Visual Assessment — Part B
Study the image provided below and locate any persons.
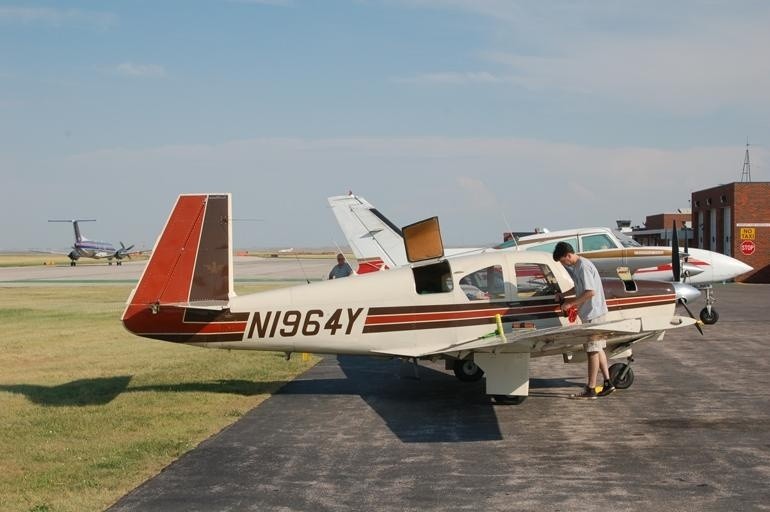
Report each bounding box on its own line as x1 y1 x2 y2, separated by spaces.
329 254 352 280
553 241 615 400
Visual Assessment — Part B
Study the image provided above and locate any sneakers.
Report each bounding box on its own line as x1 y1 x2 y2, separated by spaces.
574 386 597 400
598 379 615 396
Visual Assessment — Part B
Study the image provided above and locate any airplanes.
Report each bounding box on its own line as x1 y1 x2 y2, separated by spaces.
34 219 152 266
